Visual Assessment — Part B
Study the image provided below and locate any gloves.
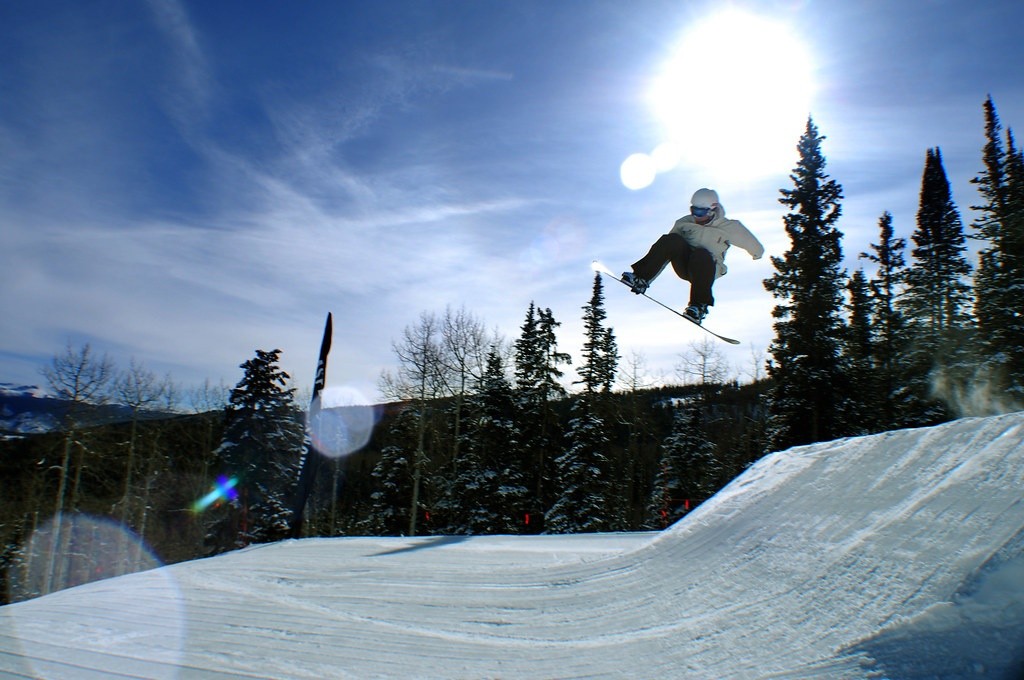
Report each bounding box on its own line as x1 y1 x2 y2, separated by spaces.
752 248 762 260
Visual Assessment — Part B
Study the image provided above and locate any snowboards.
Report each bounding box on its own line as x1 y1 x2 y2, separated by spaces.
593 261 740 347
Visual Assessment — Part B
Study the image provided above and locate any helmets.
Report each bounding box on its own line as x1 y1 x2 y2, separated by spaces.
691 188 719 209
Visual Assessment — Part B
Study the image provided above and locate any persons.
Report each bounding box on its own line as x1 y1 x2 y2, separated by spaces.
621 188 764 324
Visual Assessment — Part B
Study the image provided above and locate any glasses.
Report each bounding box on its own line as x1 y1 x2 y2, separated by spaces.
690 207 712 217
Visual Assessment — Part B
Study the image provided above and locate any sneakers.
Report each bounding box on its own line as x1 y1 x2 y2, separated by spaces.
683 303 708 324
622 272 649 294
